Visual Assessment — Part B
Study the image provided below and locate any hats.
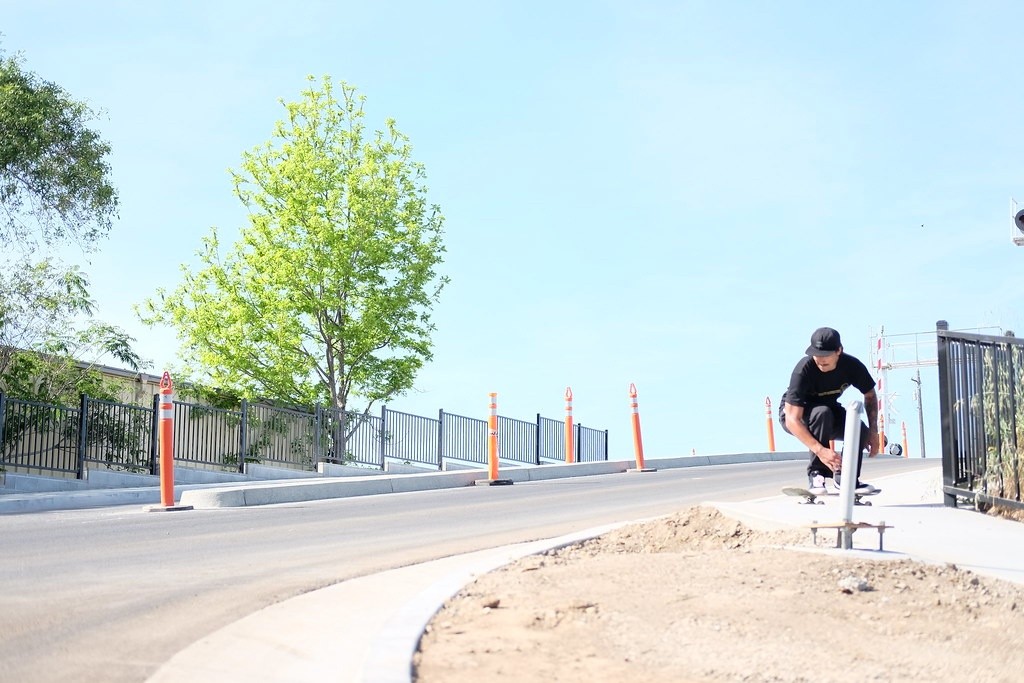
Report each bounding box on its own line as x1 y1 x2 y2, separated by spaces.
805 327 840 356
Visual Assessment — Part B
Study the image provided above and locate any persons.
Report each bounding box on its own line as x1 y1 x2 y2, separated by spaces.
779 327 881 494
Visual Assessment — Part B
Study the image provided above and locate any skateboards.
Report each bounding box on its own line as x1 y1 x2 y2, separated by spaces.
781 486 882 506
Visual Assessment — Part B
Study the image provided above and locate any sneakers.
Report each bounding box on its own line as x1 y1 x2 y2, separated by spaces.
809 471 828 494
834 479 875 494
